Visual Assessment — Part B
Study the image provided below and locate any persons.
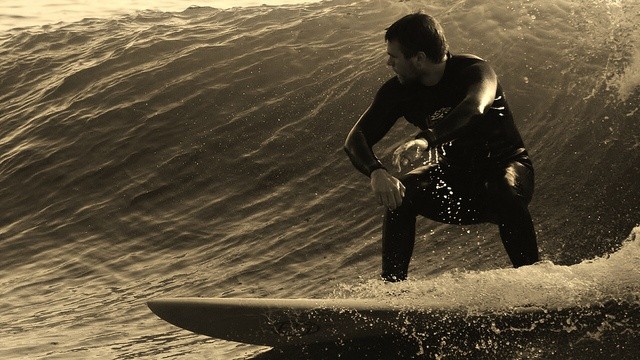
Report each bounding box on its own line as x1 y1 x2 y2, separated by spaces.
344 13 539 282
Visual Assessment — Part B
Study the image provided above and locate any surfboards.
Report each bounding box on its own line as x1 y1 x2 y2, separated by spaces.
147 298 640 360
